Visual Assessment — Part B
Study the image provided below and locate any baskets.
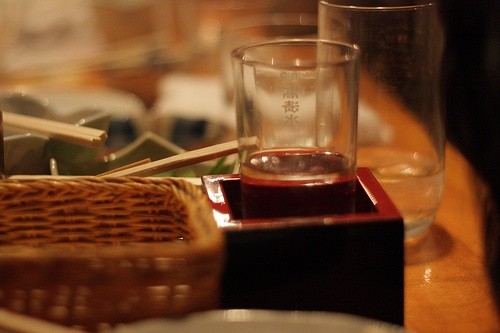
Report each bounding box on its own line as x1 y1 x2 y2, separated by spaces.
0 174 225 333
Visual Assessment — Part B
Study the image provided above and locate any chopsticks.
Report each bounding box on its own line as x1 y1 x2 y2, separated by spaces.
99 136 259 177
0 110 108 147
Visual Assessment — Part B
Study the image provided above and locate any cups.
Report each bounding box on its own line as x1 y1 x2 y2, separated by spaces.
315 1 445 237
231 38 361 219
